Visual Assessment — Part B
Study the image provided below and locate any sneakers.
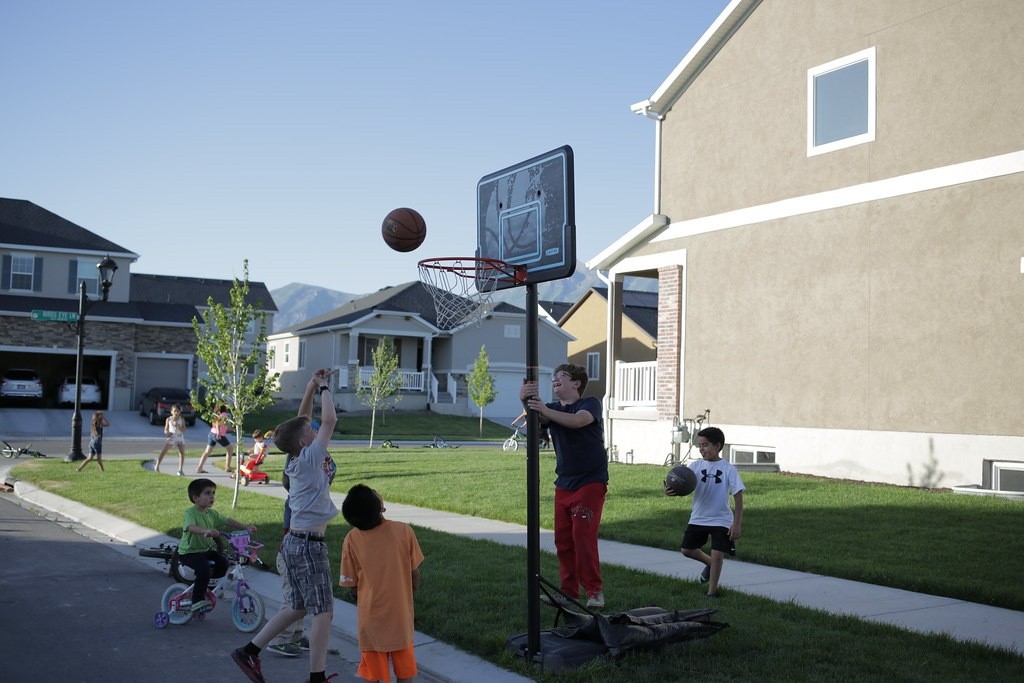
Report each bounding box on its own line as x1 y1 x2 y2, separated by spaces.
295 638 310 650
266 644 300 656
231 647 265 683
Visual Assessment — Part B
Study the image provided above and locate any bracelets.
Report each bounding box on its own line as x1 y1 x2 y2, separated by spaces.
319 386 330 394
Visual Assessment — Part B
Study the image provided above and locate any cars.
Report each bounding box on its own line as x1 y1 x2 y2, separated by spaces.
140 386 196 426
56 376 104 409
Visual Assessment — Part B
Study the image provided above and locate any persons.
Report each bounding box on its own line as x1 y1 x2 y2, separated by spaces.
177 479 257 613
197 399 233 472
512 410 550 448
76 411 110 471
663 427 746 598
229 368 340 683
519 364 610 608
154 404 185 475
246 429 269 469
339 484 425 683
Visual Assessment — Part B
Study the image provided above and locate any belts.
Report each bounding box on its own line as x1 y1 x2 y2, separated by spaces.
290 531 323 541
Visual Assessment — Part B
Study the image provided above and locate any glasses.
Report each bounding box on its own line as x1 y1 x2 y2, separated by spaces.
552 372 572 381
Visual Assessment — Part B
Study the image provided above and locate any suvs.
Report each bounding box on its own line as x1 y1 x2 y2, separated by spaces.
0 368 45 408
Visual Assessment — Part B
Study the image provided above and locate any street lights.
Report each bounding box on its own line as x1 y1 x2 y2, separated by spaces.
66 253 121 461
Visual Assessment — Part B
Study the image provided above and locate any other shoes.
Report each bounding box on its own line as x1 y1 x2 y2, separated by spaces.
226 469 232 472
707 590 720 599
587 592 605 608
197 471 208 473
700 565 711 584
540 595 579 606
177 470 185 476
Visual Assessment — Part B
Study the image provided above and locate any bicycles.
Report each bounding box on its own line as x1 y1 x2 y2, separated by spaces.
153 528 267 634
502 424 549 451
380 438 399 449
137 540 251 578
422 432 461 449
0 440 48 459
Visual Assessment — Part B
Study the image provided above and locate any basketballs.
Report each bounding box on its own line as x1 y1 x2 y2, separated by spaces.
665 465 697 496
381 207 427 252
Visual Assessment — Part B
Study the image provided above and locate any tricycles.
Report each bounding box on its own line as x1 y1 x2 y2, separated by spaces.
230 429 277 487
